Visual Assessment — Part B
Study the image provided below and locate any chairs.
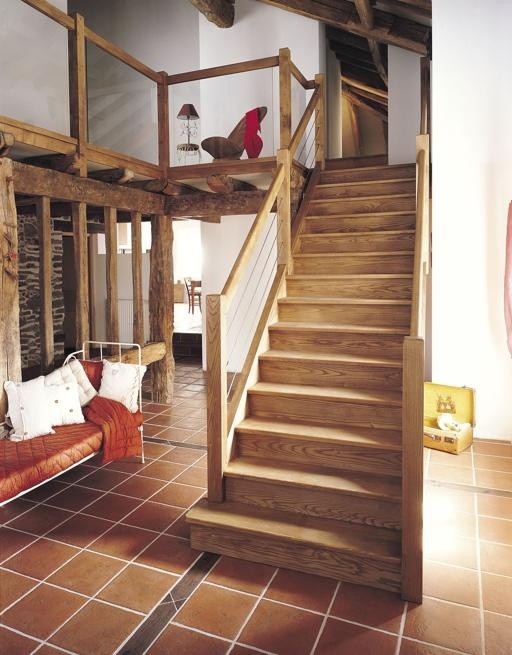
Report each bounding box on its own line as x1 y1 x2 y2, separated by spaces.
201 106 267 160
183 276 201 314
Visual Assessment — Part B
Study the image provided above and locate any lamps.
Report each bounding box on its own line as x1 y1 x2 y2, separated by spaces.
176 104 200 144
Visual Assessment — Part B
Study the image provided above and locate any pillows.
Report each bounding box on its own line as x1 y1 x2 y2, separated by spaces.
3 358 148 444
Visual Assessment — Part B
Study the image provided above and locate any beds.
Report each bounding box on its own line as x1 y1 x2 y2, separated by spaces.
0 341 145 507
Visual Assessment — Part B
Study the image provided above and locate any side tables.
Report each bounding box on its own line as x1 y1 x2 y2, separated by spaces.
175 143 201 166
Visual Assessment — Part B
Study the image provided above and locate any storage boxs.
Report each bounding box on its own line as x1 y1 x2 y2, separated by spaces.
423 382 477 455
